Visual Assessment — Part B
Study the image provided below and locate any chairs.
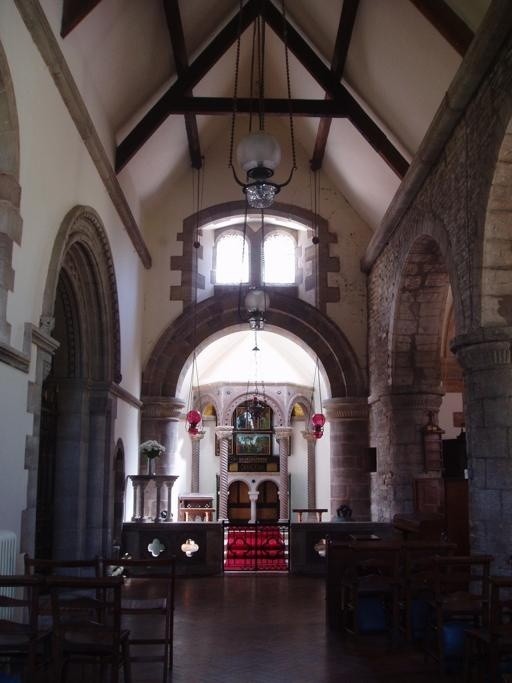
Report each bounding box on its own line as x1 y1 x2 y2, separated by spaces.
321 531 511 682
1 551 176 683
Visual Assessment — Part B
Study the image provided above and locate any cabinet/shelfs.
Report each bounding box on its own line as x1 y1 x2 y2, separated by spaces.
177 494 216 523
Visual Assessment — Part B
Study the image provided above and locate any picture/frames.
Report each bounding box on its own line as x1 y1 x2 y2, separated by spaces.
231 406 273 458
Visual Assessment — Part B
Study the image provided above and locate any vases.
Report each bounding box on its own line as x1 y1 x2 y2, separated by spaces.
146 457 159 479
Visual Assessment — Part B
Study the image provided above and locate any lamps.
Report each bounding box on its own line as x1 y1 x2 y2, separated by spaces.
245 379 271 427
227 0 299 214
184 407 207 445
235 278 273 329
300 410 328 445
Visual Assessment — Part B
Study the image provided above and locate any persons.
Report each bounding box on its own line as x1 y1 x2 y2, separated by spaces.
329 504 355 523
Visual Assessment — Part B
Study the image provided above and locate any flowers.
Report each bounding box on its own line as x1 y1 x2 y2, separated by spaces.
139 439 168 457
